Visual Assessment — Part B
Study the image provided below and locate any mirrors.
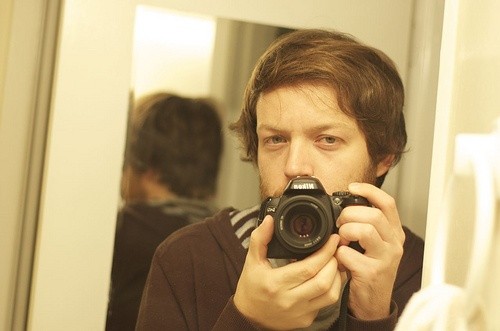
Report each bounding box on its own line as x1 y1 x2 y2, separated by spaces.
106 5 355 331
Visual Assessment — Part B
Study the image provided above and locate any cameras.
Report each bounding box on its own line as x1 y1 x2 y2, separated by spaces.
257 176 372 259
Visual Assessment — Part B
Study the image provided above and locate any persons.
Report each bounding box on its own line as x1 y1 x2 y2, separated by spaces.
135 28 425 331
105 92 237 331
